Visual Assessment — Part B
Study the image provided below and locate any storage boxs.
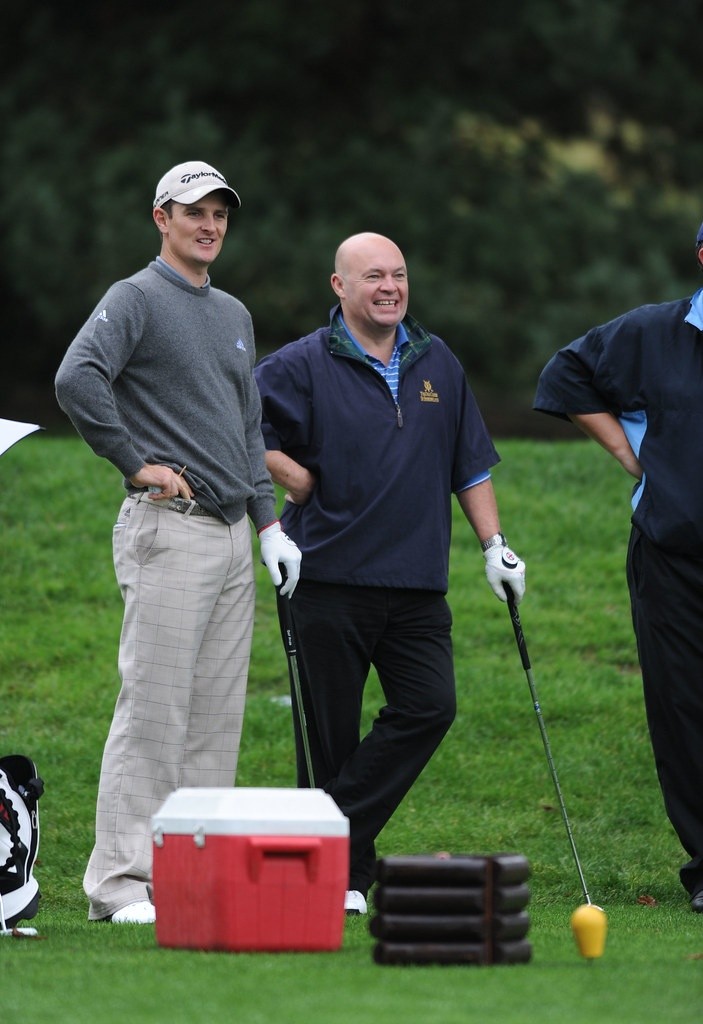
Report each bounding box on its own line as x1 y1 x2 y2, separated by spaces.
152 787 351 950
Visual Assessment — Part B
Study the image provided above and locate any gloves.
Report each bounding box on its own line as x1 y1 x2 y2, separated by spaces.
482 543 526 606
257 519 302 599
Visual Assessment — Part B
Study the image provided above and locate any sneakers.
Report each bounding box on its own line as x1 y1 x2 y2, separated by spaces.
110 901 156 924
344 889 366 915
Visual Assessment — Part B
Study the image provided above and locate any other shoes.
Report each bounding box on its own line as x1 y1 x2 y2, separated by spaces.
691 890 703 913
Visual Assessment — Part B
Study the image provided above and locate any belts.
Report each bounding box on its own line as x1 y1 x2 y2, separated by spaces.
140 494 215 516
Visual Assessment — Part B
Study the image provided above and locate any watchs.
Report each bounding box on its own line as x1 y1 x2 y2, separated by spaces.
480 533 507 552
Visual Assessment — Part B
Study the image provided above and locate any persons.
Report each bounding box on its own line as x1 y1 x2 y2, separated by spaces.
253 232 526 915
529 221 703 914
54 160 303 923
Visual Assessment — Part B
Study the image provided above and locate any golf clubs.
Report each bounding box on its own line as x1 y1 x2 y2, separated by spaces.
270 560 316 786
503 580 596 908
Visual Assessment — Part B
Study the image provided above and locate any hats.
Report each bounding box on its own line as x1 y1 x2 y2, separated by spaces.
695 221 703 247
152 160 241 209
